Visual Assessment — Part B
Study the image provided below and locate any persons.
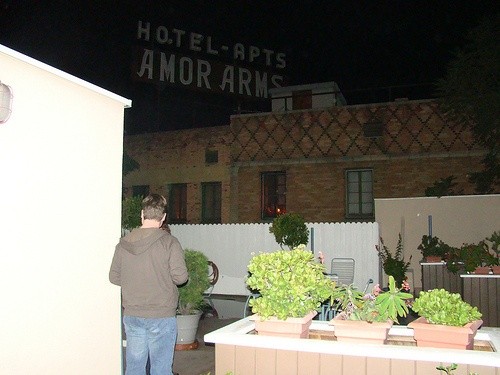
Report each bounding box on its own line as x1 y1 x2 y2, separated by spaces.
109 193 189 375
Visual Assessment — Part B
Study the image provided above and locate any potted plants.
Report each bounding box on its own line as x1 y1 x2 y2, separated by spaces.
174 250 212 350
417 234 500 276
245 244 483 350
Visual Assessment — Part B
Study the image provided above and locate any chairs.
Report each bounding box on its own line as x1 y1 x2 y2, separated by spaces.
329 257 355 288
